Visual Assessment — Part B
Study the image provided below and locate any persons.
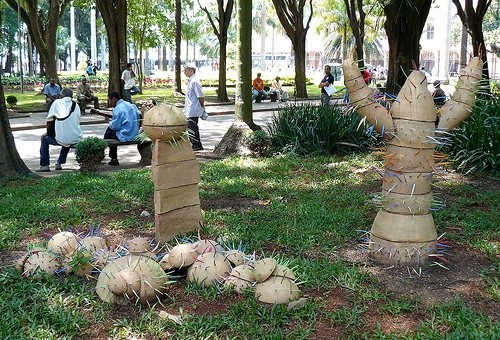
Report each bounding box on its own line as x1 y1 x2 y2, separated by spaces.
86 63 99 76
363 66 384 86
76 77 101 115
319 65 334 104
183 62 204 152
273 76 290 101
121 63 139 103
252 73 268 101
35 89 82 172
420 66 426 76
104 90 143 166
431 79 446 107
44 78 62 113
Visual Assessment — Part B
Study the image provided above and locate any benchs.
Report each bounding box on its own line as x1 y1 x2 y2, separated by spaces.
73 100 92 104
255 93 277 103
69 138 138 159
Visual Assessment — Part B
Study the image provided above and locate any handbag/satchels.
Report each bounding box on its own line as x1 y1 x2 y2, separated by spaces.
47 116 55 137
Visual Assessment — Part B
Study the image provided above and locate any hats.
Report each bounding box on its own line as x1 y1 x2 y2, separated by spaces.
433 79 441 87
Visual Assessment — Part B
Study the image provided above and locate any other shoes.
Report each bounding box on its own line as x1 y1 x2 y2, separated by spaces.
35 165 50 172
55 163 62 170
82 111 85 115
192 145 203 151
109 158 119 165
